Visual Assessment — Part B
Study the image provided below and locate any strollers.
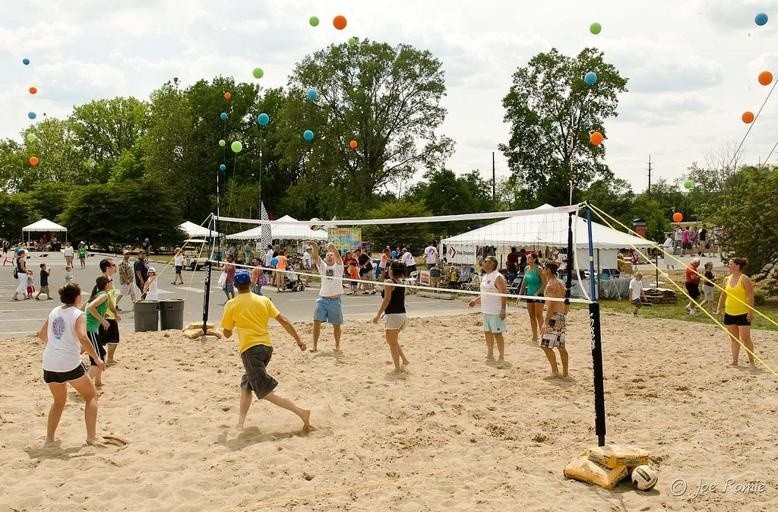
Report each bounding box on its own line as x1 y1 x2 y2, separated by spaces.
278 264 305 293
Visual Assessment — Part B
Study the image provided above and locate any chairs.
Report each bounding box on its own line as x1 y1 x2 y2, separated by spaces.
224 258 524 300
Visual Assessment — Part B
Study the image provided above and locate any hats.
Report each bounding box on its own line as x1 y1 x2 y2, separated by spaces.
123 250 130 255
233 269 250 283
147 267 156 273
173 247 182 253
80 241 86 244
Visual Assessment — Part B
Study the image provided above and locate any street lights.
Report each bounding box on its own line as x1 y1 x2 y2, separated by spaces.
669 206 675 216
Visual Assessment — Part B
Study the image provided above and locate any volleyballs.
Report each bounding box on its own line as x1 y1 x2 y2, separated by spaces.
309 217 321 231
631 464 659 491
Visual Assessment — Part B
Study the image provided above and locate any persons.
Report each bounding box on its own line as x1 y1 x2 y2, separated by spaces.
515 252 549 341
467 255 509 364
519 248 527 273
219 268 311 431
99 258 123 365
80 275 116 388
664 224 730 259
0 230 90 303
304 239 345 353
218 241 475 295
170 247 185 286
693 261 717 315
133 249 149 299
626 272 648 316
37 281 106 448
506 247 517 276
681 258 703 318
141 267 158 301
539 259 571 380
114 249 138 311
369 261 410 373
716 256 756 367
142 237 152 257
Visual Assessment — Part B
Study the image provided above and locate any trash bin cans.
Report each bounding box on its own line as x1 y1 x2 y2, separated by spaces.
159 299 185 331
133 299 159 332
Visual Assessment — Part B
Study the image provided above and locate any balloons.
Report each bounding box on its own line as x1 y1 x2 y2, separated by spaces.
257 113 270 126
252 68 264 80
219 164 227 171
308 16 320 27
349 140 358 149
223 91 232 101
589 22 601 35
672 212 683 223
332 14 347 31
26 134 38 144
684 180 692 189
754 13 769 27
742 111 754 124
29 156 39 166
27 112 36 120
218 140 226 147
220 113 229 120
590 132 603 146
584 72 597 85
303 130 314 142
306 89 317 101
757 71 773 86
22 58 30 66
231 140 242 153
28 86 37 95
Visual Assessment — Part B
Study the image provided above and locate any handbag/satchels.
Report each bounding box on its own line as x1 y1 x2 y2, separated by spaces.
218 271 228 289
258 274 267 285
